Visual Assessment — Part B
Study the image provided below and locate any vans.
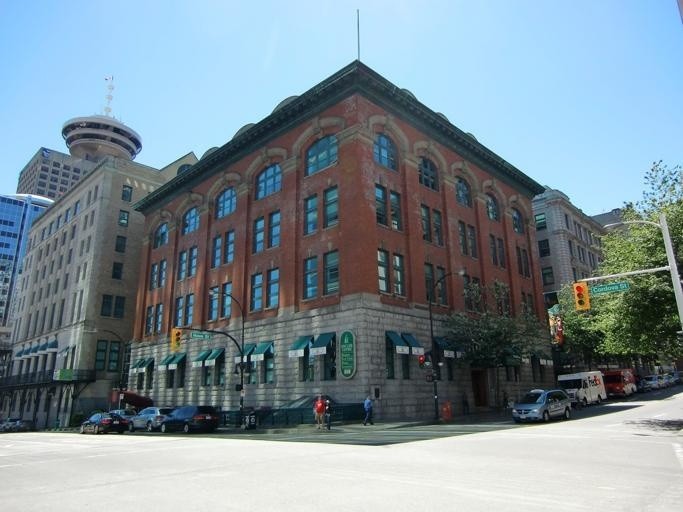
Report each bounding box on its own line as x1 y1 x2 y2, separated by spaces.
600 368 638 398
557 370 607 407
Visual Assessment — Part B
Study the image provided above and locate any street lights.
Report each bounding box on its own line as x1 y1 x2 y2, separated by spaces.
208 289 246 428
602 214 682 329
428 269 467 423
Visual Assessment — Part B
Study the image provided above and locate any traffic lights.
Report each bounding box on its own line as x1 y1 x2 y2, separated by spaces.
573 281 591 312
418 355 424 369
170 328 183 351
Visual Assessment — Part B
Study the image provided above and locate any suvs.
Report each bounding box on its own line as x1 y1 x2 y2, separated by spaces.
511 388 573 421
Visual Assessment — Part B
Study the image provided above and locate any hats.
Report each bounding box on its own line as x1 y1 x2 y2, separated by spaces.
326 400 330 403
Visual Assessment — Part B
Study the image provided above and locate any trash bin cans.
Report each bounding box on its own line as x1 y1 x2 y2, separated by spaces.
245 415 256 430
55 420 60 429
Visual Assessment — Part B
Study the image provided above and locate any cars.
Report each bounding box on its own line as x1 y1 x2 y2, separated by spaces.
78 403 219 435
0 417 35 432
634 370 682 392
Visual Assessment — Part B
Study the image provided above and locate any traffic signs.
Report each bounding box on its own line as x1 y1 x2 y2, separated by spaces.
591 281 630 293
191 332 210 339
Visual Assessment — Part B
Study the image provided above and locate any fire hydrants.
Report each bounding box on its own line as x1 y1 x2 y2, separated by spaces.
443 399 452 422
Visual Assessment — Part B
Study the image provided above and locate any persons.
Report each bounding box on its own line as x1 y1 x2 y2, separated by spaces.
363 395 373 425
15 418 20 432
549 395 555 404
637 373 672 394
574 387 582 410
312 394 325 430
659 367 665 374
324 399 333 430
461 391 470 415
